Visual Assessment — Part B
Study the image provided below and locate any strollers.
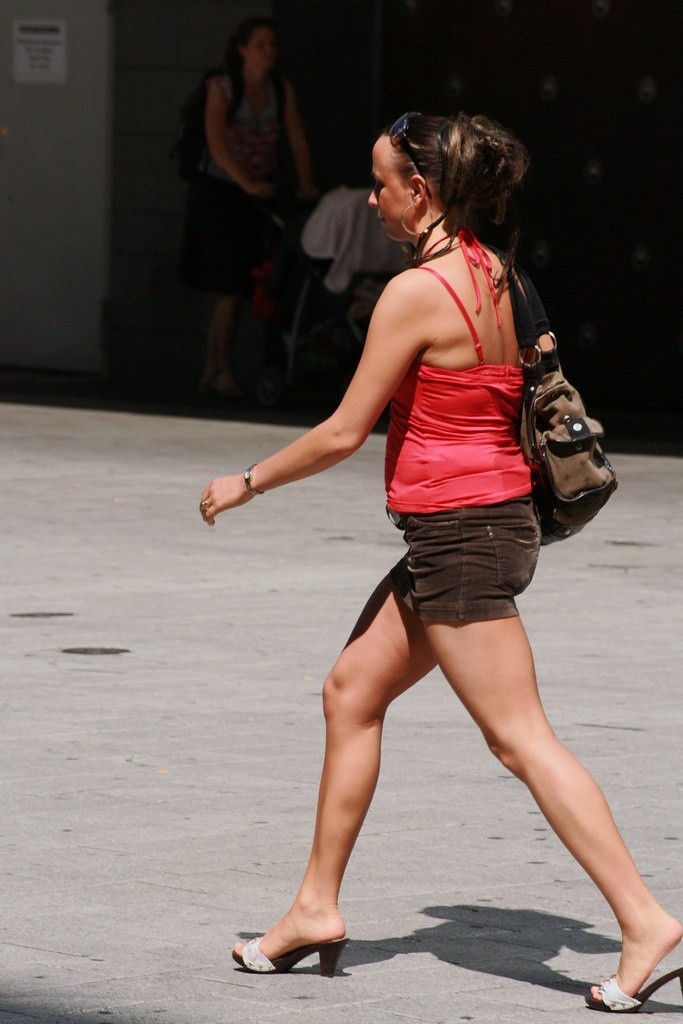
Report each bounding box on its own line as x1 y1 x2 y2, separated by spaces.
253 176 396 408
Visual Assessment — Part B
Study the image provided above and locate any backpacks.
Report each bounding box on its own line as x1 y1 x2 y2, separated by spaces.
175 69 287 177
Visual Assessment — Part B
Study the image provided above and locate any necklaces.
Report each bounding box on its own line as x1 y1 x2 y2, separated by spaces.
421 232 458 260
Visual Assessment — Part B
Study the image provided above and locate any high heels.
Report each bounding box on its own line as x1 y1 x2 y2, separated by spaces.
231 919 352 978
583 965 683 1014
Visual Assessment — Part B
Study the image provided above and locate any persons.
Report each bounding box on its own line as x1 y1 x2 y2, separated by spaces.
194 14 319 400
200 109 683 1013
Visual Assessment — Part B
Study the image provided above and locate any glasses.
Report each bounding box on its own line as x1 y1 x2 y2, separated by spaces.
389 111 432 200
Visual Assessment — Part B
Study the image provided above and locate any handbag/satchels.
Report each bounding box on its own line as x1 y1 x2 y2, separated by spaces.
480 238 616 543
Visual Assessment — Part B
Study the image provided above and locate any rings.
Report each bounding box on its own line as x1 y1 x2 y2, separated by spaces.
201 500 209 511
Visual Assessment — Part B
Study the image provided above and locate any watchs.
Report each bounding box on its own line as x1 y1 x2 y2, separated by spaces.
244 464 265 495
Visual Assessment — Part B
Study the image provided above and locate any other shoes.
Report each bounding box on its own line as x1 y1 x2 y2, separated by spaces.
211 366 246 405
